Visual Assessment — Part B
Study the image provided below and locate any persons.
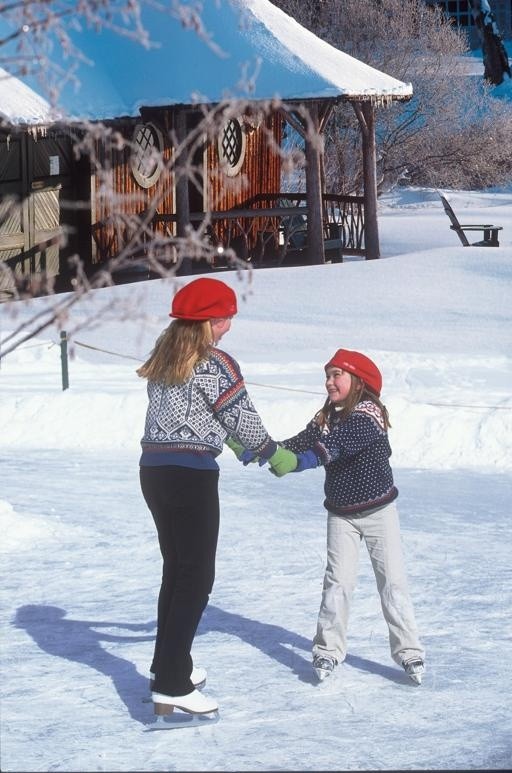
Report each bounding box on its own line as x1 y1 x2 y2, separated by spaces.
238 349 428 674
135 274 298 715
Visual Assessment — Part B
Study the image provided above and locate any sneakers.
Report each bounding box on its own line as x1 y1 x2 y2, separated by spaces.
312 653 336 671
401 655 423 676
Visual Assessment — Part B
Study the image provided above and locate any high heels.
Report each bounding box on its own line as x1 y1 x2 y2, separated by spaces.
149 666 208 691
152 690 218 717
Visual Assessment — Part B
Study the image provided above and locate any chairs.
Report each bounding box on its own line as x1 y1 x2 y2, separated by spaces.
274 197 343 263
441 196 503 247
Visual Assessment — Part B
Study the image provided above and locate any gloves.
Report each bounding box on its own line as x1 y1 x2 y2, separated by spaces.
269 446 319 477
226 435 268 467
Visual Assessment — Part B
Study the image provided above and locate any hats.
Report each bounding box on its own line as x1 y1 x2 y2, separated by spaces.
324 349 382 397
168 278 238 323
